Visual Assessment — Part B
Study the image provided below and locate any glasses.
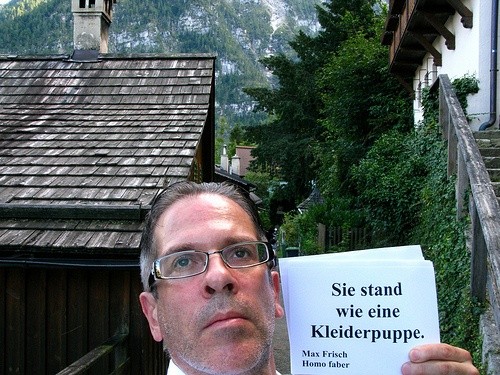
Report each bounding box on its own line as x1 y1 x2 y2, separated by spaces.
148 240 274 287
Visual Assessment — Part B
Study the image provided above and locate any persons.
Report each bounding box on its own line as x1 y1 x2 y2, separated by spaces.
139 179 481 375
